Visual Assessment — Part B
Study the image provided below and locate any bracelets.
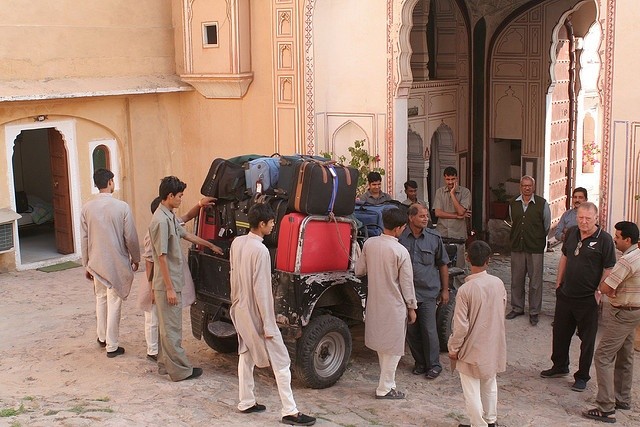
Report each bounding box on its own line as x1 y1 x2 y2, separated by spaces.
198 199 202 207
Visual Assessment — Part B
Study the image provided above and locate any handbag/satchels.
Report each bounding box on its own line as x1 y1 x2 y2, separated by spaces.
218 155 269 200
200 159 226 198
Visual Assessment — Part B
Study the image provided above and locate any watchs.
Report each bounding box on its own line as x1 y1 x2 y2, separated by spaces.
596 289 602 294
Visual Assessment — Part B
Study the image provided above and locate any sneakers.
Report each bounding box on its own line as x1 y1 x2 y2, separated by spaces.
97 337 106 346
425 362 442 379
282 412 316 426
107 346 125 358
541 365 569 378
147 354 158 362
571 377 588 391
183 368 203 380
240 402 266 413
412 364 425 375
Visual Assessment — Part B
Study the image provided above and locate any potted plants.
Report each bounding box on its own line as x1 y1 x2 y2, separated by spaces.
489 183 510 218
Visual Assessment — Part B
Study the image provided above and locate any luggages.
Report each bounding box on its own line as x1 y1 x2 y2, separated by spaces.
203 239 233 259
290 158 359 216
241 157 281 197
231 198 251 236
276 213 351 273
273 155 328 200
265 199 293 247
217 199 236 238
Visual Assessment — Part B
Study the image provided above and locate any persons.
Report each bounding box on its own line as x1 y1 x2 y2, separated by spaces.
401 182 431 225
143 198 216 362
555 186 586 244
359 172 391 205
356 208 418 400
150 175 223 381
540 203 616 392
230 204 316 425
580 222 640 422
448 241 508 427
81 169 141 359
505 175 551 325
399 202 449 377
434 167 472 291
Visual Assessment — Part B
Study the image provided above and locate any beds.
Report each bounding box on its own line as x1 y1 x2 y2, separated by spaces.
16 194 53 233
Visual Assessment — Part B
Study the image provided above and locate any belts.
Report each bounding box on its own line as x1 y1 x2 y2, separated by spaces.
610 303 640 310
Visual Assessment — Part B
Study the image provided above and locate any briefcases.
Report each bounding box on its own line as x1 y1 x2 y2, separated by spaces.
196 205 217 251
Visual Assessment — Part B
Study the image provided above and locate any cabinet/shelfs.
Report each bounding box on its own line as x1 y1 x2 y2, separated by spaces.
14 140 24 192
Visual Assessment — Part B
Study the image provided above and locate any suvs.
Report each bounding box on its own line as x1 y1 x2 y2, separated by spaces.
188 200 465 389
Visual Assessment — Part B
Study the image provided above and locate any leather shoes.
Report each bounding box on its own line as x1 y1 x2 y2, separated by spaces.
529 314 539 326
505 310 525 319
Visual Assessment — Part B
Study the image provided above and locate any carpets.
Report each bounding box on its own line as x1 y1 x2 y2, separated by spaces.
36 260 83 273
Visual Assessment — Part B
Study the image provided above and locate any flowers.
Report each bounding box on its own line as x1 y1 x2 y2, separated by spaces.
320 138 385 198
582 141 601 167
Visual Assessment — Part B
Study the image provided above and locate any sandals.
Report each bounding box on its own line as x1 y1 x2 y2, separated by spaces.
614 399 630 410
375 388 406 399
582 407 616 423
